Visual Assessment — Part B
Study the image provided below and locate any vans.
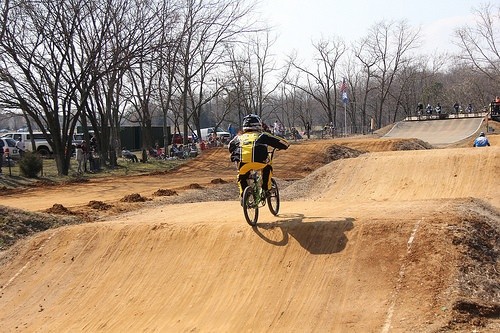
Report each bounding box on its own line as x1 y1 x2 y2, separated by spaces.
3 133 55 159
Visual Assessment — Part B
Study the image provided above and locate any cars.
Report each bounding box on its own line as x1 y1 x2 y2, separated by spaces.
171 132 193 144
1 137 18 159
1 129 7 132
19 127 26 131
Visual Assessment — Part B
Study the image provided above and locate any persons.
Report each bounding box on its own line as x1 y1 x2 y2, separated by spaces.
207 123 238 148
453 101 459 112
228 114 290 208
75 137 100 173
473 131 491 147
16 137 26 155
481 106 488 112
496 96 500 102
0 139 5 173
416 100 442 114
465 103 473 112
121 140 199 163
273 120 312 140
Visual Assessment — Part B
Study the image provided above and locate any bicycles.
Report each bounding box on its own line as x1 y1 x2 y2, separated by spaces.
416 106 486 120
243 146 279 225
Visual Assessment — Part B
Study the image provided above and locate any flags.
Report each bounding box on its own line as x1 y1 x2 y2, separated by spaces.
341 82 350 103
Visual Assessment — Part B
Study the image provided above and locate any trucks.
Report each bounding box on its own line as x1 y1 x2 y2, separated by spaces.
193 127 231 143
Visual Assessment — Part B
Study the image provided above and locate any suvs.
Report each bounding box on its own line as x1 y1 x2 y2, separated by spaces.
70 135 89 154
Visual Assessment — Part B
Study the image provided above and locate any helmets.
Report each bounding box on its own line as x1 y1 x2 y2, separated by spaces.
479 132 484 137
243 115 262 126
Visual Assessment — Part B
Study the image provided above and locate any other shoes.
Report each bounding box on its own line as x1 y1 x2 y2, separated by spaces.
264 187 275 197
241 198 243 206
